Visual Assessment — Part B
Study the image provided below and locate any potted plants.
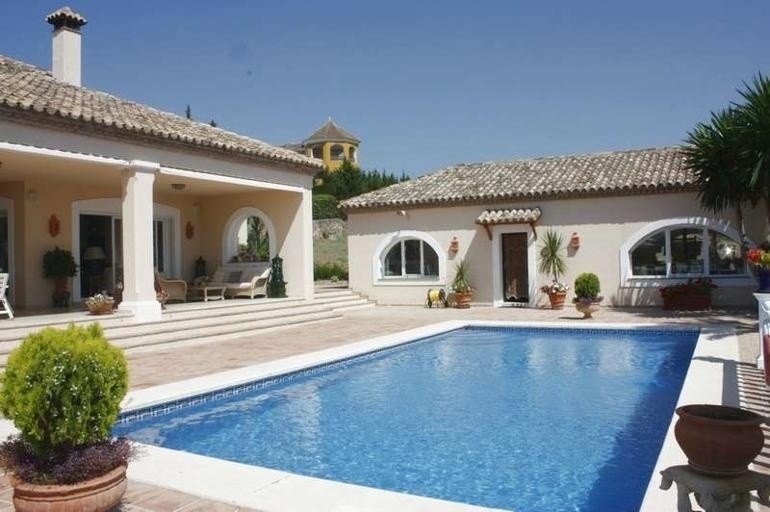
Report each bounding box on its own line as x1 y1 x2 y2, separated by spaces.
41 246 81 307
572 272 604 319
539 229 569 310
452 260 476 309
0 321 132 511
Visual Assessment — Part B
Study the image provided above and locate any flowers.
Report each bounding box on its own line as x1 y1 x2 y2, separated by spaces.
659 276 718 298
744 247 770 272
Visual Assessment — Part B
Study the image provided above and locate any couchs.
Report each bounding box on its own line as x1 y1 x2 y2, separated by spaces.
193 266 273 300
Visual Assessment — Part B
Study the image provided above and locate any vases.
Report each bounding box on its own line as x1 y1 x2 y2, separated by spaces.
674 403 766 476
752 270 770 293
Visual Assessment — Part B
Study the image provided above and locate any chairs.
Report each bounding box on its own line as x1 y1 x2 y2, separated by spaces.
155 272 188 303
1 272 14 320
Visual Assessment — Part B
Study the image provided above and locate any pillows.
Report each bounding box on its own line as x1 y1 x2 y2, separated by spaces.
212 271 242 283
